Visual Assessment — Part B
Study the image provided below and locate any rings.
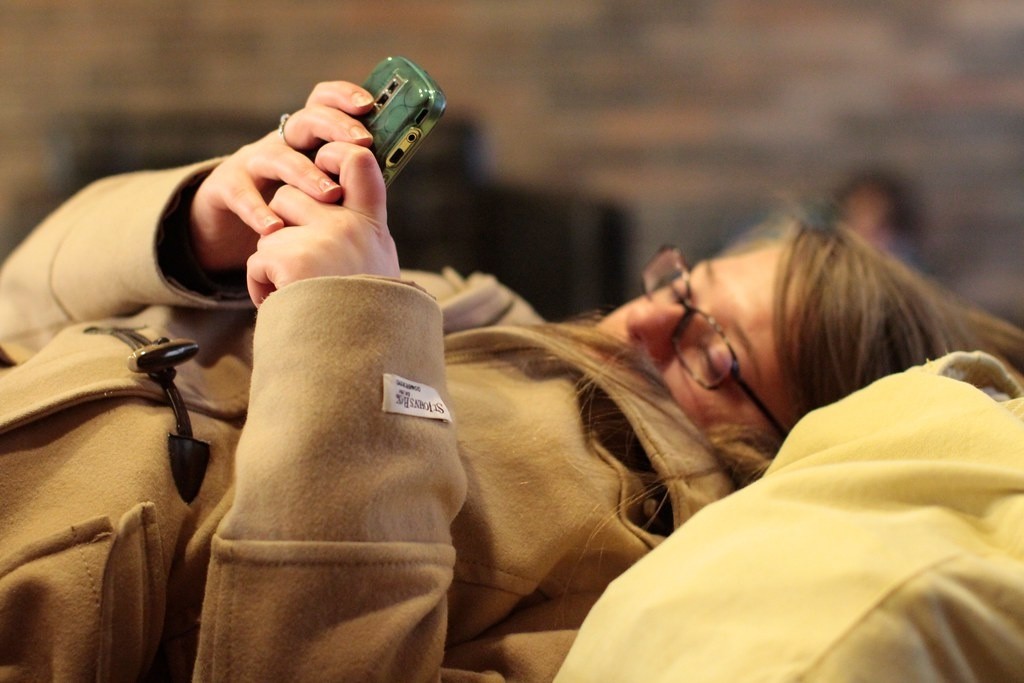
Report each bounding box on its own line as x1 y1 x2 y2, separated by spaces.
279 113 289 143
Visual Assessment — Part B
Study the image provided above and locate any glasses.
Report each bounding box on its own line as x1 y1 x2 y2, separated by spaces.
633 245 788 442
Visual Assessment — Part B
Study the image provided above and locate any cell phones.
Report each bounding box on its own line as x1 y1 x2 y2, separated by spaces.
280 53 449 205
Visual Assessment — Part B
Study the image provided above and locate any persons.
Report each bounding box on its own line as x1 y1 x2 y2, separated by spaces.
0 79 1024 683
